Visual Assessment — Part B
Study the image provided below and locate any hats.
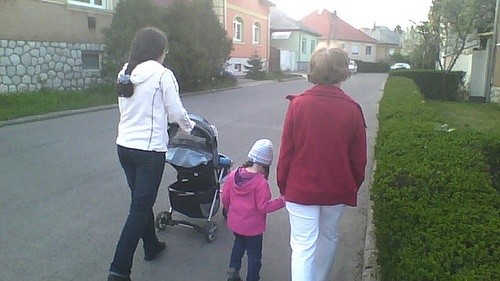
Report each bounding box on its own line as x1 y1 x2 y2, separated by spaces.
247 139 274 166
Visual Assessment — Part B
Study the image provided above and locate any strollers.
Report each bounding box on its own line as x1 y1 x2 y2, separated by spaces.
156 115 234 243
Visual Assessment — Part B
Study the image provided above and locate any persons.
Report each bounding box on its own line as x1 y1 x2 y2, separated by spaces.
276 42 367 281
221 139 286 281
106 27 196 281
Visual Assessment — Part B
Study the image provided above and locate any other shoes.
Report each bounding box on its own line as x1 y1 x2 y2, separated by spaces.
144 242 165 260
228 268 242 280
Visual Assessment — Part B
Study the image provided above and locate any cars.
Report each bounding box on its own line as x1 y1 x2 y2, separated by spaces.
390 63 411 70
348 60 357 73
